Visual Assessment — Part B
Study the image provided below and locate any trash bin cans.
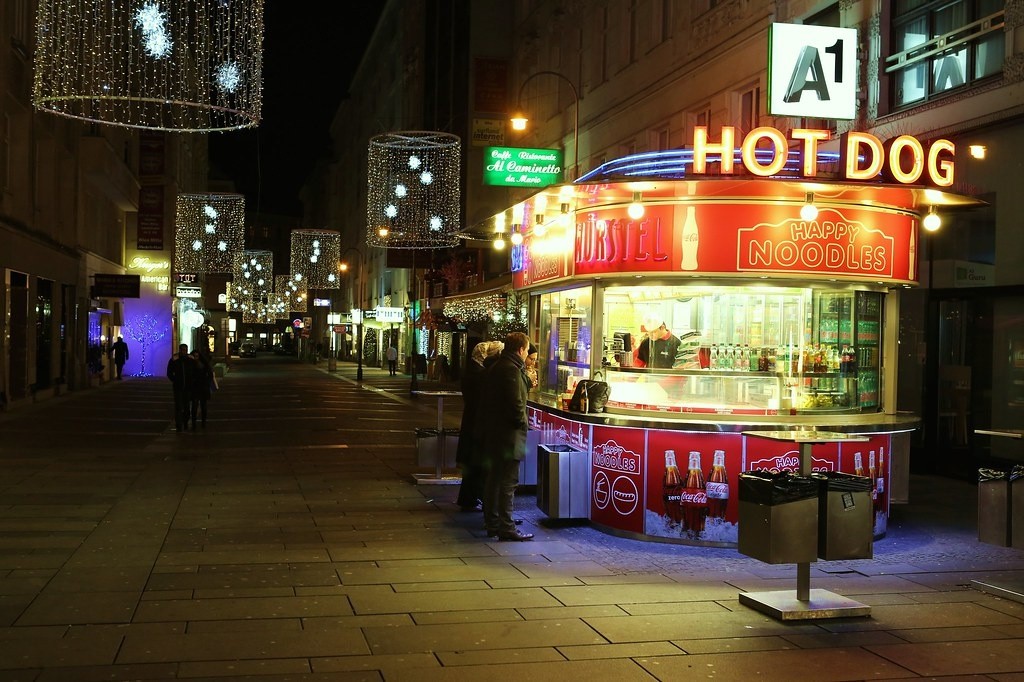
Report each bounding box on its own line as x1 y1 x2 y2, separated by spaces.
811 471 872 560
736 472 819 564
977 465 1024 548
537 443 590 520
444 428 459 468
414 428 438 466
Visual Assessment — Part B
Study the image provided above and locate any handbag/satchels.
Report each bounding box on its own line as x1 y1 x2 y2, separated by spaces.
568 371 610 413
208 371 219 392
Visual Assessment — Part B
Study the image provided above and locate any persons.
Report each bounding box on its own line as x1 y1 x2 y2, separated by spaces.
167 343 194 433
109 337 129 380
386 344 397 376
615 309 687 401
456 333 538 542
190 349 211 429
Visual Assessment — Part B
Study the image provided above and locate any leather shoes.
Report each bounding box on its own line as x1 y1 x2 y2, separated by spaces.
499 527 533 541
487 528 501 536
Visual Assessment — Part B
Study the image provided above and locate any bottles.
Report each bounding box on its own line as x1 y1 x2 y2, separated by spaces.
580 382 589 414
802 387 847 409
681 452 707 540
663 450 683 527
704 450 730 524
855 445 885 509
709 343 856 374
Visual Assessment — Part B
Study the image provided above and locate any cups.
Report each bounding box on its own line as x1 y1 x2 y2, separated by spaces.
568 349 577 361
562 399 571 410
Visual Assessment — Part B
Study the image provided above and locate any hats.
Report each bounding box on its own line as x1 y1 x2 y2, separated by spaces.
472 341 504 358
642 303 664 331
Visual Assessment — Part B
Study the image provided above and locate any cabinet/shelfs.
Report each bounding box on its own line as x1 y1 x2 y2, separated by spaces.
539 359 862 417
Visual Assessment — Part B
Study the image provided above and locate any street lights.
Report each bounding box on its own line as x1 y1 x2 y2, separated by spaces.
341 247 363 381
511 70 579 181
378 214 418 401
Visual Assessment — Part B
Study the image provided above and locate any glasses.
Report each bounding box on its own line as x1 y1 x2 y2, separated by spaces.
528 356 538 361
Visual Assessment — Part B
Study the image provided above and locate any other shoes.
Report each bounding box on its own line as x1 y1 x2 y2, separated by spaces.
457 499 483 512
175 423 188 432
202 418 208 424
192 424 196 430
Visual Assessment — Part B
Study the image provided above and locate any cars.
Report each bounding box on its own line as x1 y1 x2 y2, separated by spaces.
273 343 296 356
229 342 240 354
238 343 256 358
